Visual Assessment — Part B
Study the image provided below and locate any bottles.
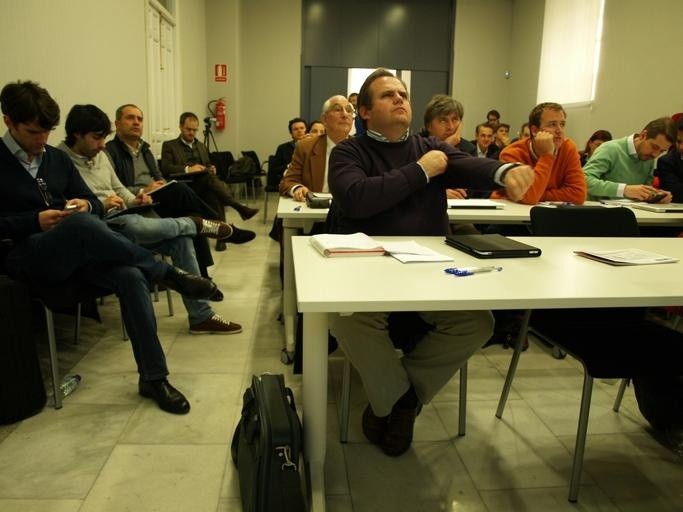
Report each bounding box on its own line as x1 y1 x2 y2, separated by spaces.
47 374 82 404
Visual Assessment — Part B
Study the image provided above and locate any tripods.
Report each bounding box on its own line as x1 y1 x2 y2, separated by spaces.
202 127 218 154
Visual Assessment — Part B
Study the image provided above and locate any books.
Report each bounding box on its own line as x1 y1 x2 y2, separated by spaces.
442 232 542 261
309 231 388 259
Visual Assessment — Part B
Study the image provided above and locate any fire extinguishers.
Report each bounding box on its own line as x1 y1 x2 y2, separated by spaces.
207 97 226 130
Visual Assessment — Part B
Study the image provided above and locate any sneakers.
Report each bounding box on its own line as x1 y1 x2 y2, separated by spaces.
362 403 388 445
191 216 233 239
377 399 423 457
189 312 241 335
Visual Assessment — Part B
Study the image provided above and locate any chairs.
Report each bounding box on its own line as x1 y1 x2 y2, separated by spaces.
240 151 270 202
72 252 174 350
92 283 157 320
341 313 471 444
494 208 683 503
210 153 253 212
2 281 129 411
264 157 271 222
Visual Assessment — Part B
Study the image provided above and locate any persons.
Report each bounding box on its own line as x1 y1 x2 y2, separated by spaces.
656 112 683 204
482 102 587 352
577 129 613 166
0 80 218 415
323 68 537 458
278 94 355 202
348 92 364 136
416 94 480 235
582 116 678 205
104 103 256 302
484 109 502 144
510 122 531 145
284 119 326 177
54 103 243 335
470 123 501 161
159 111 260 253
492 123 510 149
274 117 309 183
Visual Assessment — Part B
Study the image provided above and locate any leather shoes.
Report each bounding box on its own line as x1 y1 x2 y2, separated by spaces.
239 206 259 221
163 266 217 300
216 239 226 251
208 278 223 302
139 376 191 414
223 223 256 244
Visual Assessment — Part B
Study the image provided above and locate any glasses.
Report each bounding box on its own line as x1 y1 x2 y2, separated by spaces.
489 118 497 121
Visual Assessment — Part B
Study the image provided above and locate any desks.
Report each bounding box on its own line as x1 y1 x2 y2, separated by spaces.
290 235 682 511
276 194 683 359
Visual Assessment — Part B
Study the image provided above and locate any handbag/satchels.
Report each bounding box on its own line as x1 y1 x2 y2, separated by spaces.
232 373 312 512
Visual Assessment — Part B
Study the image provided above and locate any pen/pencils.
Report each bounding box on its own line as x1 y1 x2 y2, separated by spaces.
444 265 502 276
